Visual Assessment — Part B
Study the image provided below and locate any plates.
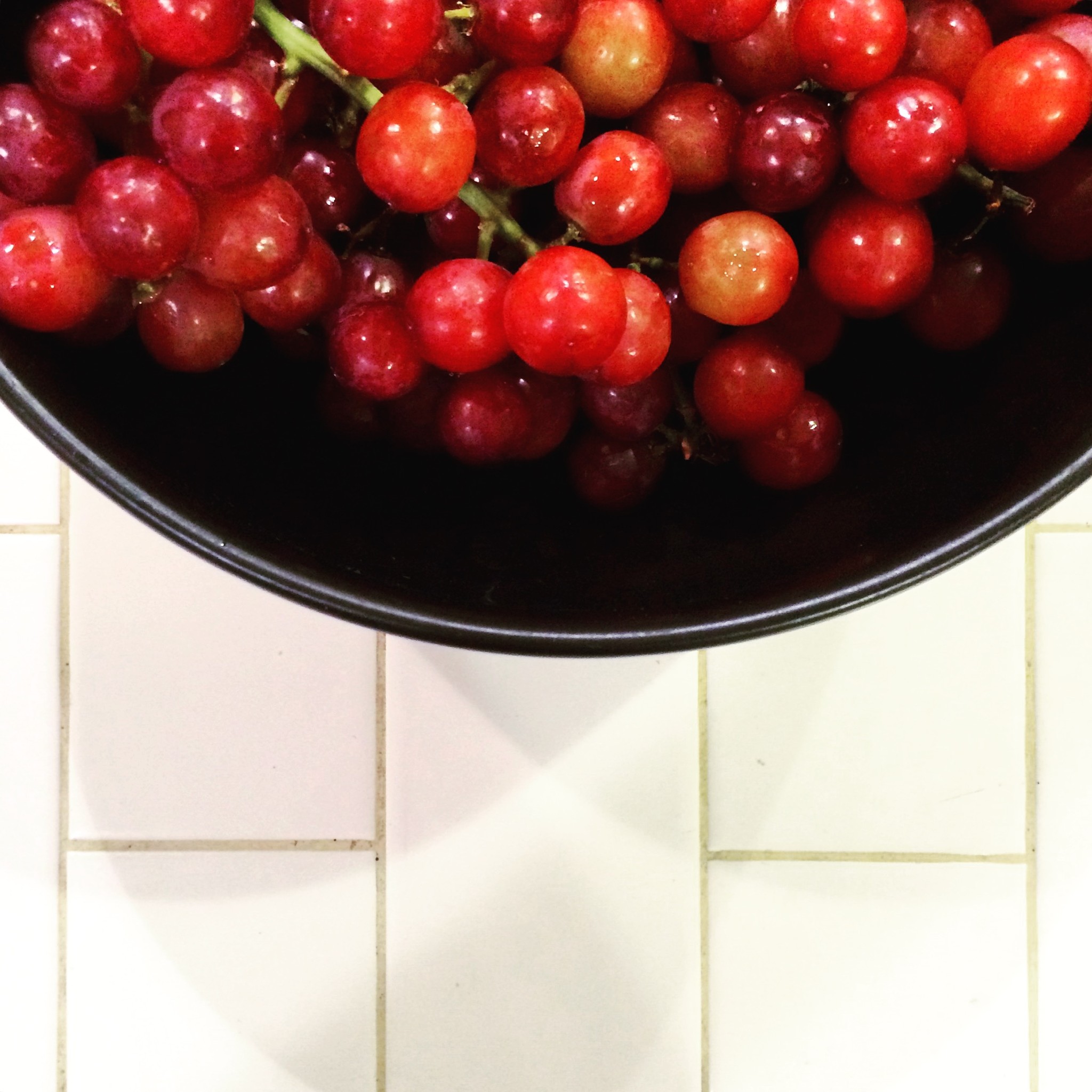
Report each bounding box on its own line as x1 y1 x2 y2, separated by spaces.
0 0 1092 657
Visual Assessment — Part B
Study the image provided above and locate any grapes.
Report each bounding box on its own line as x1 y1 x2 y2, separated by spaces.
0 0 1092 512
